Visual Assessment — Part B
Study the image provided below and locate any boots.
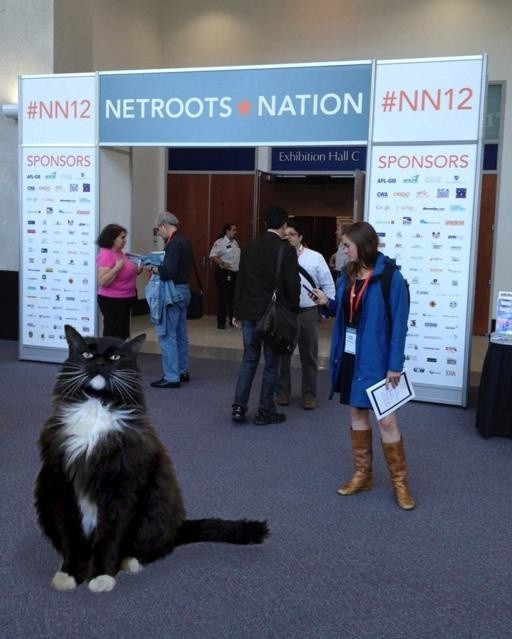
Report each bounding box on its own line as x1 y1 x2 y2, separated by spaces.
380 436 416 510
336 427 373 496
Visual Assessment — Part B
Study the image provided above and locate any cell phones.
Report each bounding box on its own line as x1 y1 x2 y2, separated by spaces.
303 284 320 299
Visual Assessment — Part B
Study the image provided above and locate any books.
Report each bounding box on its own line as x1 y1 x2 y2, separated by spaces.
124 251 142 269
150 251 166 258
365 369 416 422
490 290 512 346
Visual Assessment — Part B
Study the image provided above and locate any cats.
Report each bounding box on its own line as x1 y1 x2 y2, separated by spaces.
32 324 272 595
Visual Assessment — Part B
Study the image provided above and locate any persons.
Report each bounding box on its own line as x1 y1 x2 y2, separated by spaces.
274 224 337 411
204 219 241 331
227 205 296 423
95 224 142 340
330 222 354 275
305 220 418 509
144 211 194 390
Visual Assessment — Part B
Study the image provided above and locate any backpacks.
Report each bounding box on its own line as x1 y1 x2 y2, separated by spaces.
381 258 411 327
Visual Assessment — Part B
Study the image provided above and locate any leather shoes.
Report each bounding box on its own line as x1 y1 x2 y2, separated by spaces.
275 394 290 406
180 372 191 383
303 397 317 410
254 409 286 426
231 404 246 423
150 377 181 388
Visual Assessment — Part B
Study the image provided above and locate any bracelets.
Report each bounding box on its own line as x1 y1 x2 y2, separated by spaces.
149 265 154 272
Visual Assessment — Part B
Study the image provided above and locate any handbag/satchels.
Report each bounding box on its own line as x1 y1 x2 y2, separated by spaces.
186 288 205 320
254 293 301 358
